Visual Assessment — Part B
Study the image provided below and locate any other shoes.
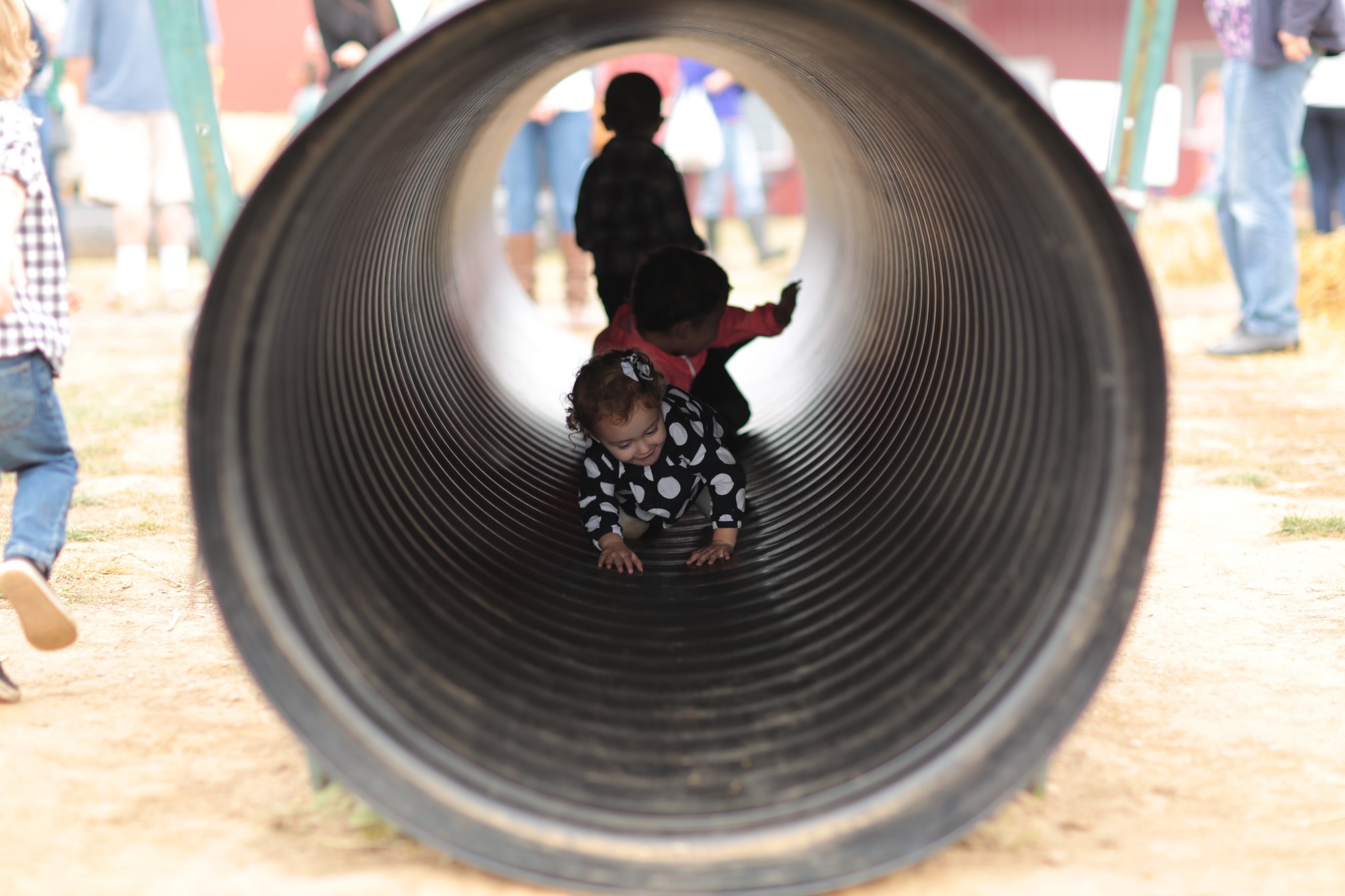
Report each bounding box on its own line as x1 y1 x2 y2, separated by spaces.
0 669 21 704
0 557 78 651
1207 331 1298 357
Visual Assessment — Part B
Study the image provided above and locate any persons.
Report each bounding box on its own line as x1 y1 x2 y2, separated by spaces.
62 0 225 289
574 70 707 326
313 0 400 82
593 53 700 218
1301 51 1345 234
25 19 73 267
1202 0 1345 354
501 66 596 308
565 345 747 574
678 55 786 258
0 0 81 702
594 245 802 431
